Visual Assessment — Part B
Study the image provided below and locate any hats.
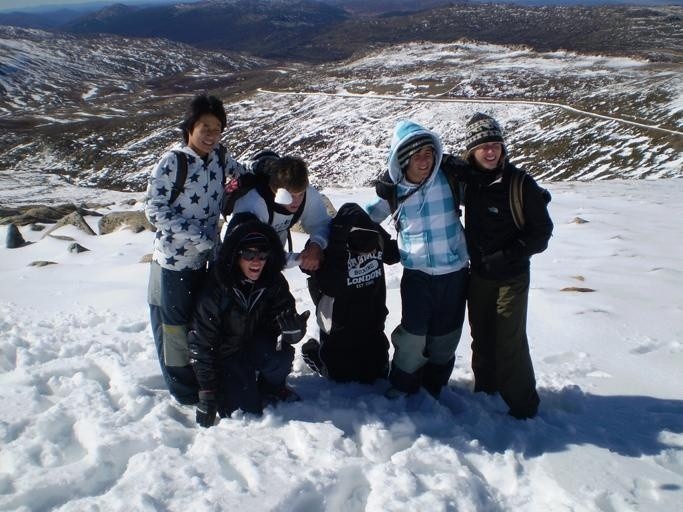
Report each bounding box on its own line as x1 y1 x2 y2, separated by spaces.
465 112 508 162
238 234 269 249
398 135 437 174
348 230 378 255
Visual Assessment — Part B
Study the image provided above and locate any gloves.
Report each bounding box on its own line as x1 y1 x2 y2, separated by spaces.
481 250 509 274
197 389 217 428
276 309 309 343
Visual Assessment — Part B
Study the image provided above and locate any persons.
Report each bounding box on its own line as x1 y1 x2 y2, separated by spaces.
299 202 400 385
184 211 310 428
232 155 333 272
376 112 553 420
362 118 469 399
145 94 250 405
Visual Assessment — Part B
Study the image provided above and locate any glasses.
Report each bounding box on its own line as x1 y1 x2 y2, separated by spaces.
239 248 270 261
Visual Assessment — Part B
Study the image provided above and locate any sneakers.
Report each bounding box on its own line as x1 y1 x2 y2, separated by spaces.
255 378 301 402
385 384 411 398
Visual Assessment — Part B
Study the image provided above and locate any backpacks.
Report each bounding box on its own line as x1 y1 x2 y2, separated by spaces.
219 150 306 230
